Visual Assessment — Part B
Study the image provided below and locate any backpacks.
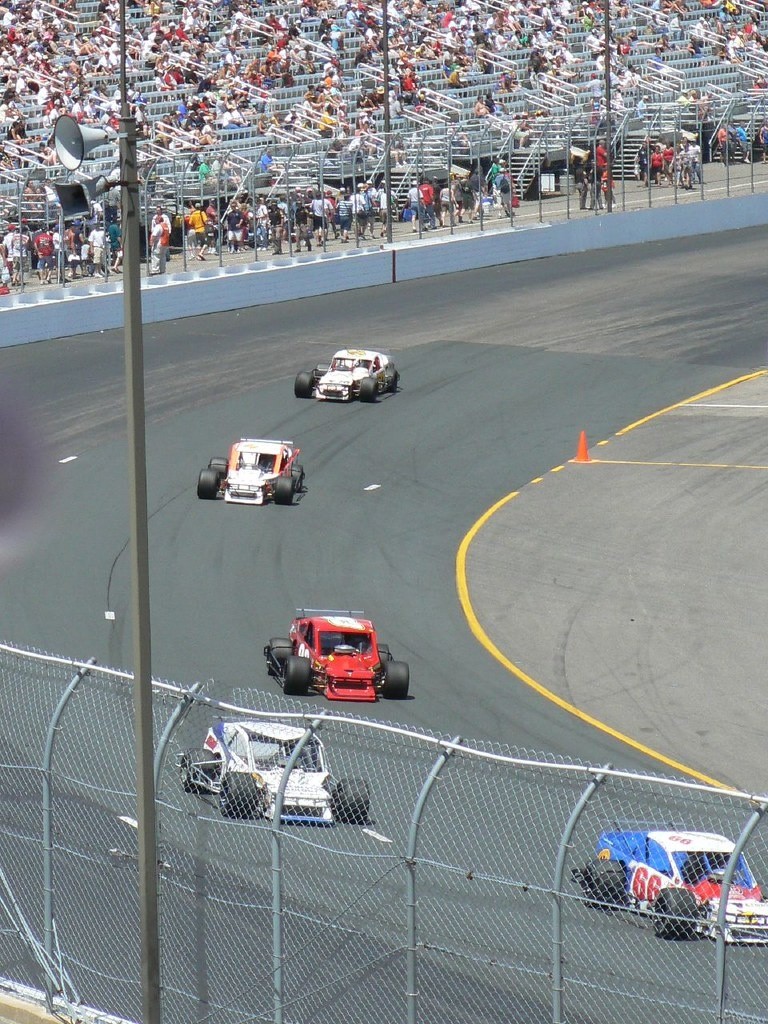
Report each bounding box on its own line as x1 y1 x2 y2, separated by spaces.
500 175 509 193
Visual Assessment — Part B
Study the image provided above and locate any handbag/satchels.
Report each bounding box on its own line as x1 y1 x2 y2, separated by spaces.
358 210 365 218
67 254 80 262
205 224 214 234
2 266 11 284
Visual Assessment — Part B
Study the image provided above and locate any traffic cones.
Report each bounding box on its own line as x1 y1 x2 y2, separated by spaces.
573 429 591 462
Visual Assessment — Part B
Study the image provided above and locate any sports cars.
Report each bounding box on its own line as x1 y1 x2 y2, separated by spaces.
293 348 400 404
571 828 768 949
180 716 371 828
197 437 305 506
264 608 409 703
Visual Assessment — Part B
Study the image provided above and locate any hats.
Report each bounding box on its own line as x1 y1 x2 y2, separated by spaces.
55 224 59 229
22 218 27 223
8 224 16 231
71 222 77 225
412 180 420 185
195 203 201 208
295 180 386 195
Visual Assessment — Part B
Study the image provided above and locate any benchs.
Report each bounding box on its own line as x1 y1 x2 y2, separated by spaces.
0 0 768 203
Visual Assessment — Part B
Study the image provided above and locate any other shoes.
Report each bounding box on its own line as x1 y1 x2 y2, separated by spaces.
412 209 515 232
11 276 26 286
36 267 122 285
184 230 388 261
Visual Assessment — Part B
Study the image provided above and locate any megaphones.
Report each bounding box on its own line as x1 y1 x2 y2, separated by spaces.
54 114 115 171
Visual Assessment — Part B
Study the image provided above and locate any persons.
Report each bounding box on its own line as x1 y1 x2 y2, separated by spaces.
150 215 169 275
227 201 245 254
494 168 515 218
0 0 767 286
188 203 210 261
